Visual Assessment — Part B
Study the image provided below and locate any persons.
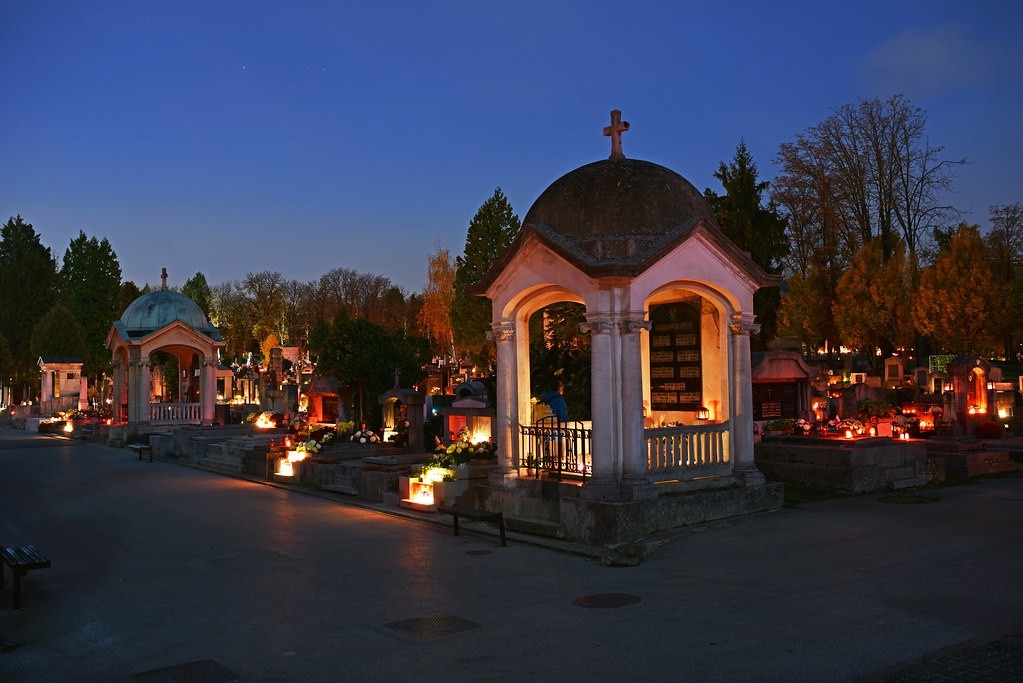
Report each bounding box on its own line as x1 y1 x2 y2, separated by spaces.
539 376 568 422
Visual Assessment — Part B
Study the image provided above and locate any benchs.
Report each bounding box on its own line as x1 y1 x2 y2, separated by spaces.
437 506 509 549
0 543 51 595
128 444 154 462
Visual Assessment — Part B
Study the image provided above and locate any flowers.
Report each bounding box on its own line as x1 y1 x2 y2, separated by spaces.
247 411 497 473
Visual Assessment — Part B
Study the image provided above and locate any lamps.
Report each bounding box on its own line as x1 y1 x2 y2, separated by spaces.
695 407 710 420
